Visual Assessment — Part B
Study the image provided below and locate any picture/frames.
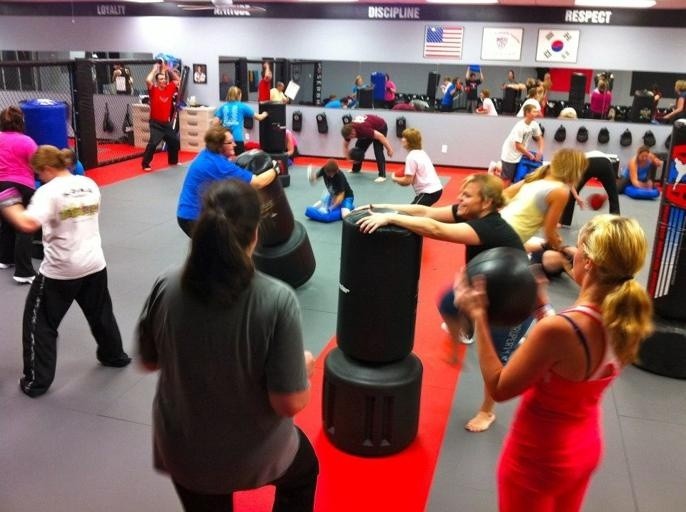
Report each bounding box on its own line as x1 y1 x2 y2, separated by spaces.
193 64 207 85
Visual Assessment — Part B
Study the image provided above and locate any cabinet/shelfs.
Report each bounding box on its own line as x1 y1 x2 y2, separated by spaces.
133 104 163 149
180 106 215 153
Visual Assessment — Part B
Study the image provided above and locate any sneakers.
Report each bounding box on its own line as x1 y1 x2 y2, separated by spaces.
13 276 35 284
0 261 10 269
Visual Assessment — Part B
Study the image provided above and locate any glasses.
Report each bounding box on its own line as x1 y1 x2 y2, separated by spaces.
220 140 233 144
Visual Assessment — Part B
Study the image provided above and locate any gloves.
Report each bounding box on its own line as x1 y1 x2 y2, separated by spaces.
0 186 22 208
307 165 314 183
271 159 281 177
318 206 332 215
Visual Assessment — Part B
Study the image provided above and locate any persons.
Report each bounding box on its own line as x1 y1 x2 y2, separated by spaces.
194 64 205 82
618 146 663 193
455 215 653 512
500 70 520 89
270 83 289 104
141 63 182 172
394 127 443 207
209 86 268 159
650 85 662 123
440 78 453 95
308 162 354 219
0 145 132 397
352 77 372 111
475 90 497 115
465 65 484 112
0 103 41 285
523 237 577 317
514 85 544 117
656 80 686 123
283 131 300 160
384 74 397 110
440 76 464 112
538 72 551 115
387 102 418 112
259 61 272 111
324 98 348 110
554 150 620 227
351 173 536 432
124 67 135 96
486 106 544 180
112 63 131 96
342 114 393 183
498 148 589 317
136 179 319 512
177 126 281 238
589 80 611 120
